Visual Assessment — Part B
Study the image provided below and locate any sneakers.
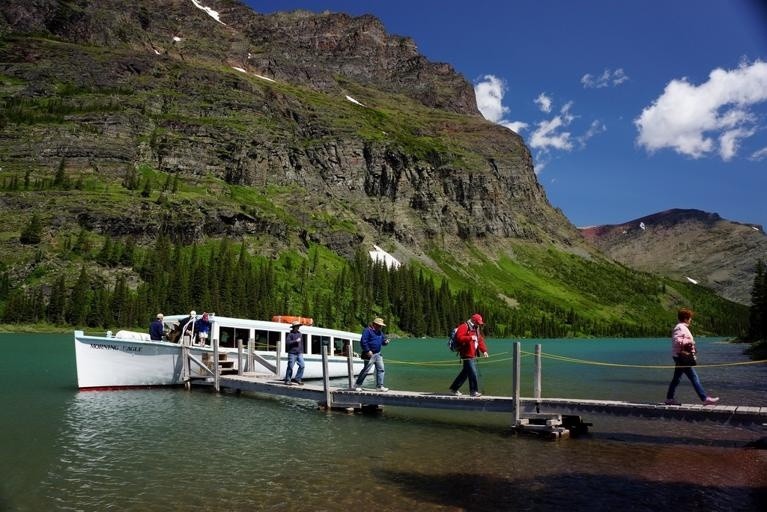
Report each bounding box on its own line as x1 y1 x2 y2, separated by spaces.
354 383 362 391
285 381 293 385
294 379 304 385
665 397 682 406
469 391 482 397
376 385 389 392
450 390 463 396
703 396 721 406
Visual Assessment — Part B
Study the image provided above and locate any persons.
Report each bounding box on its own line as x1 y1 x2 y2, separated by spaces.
177 310 196 336
449 313 489 398
148 313 166 340
285 321 305 385
353 317 389 392
191 312 209 343
664 308 721 407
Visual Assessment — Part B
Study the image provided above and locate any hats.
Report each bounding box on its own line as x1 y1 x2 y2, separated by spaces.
190 310 197 318
203 313 208 321
289 320 303 328
372 317 387 327
472 313 484 325
157 313 164 319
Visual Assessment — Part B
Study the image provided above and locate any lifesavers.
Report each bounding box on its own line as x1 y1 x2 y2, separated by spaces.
272 315 313 326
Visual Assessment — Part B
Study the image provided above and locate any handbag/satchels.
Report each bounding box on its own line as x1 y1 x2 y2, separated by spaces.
677 338 698 367
361 351 371 360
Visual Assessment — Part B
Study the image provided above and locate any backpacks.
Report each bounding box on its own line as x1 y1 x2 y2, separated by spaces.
449 321 471 352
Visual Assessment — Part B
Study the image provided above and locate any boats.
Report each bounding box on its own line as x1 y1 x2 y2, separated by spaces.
73 311 376 391
